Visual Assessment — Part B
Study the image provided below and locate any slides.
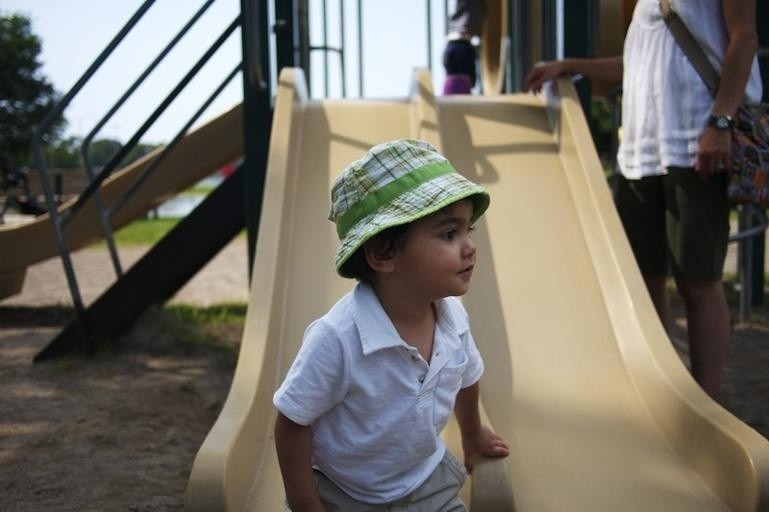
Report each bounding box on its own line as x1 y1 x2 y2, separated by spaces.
2 102 244 300
187 67 769 510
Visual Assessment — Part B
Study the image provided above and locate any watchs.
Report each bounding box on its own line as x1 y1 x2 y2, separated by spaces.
705 111 737 130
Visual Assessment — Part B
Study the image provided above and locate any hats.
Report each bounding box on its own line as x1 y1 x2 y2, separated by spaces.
328 138 490 279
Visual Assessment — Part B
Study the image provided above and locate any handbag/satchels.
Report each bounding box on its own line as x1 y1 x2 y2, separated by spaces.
727 102 769 203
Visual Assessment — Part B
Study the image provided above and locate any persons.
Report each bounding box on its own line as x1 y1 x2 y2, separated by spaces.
272 138 510 512
442 22 476 95
523 0 763 403
